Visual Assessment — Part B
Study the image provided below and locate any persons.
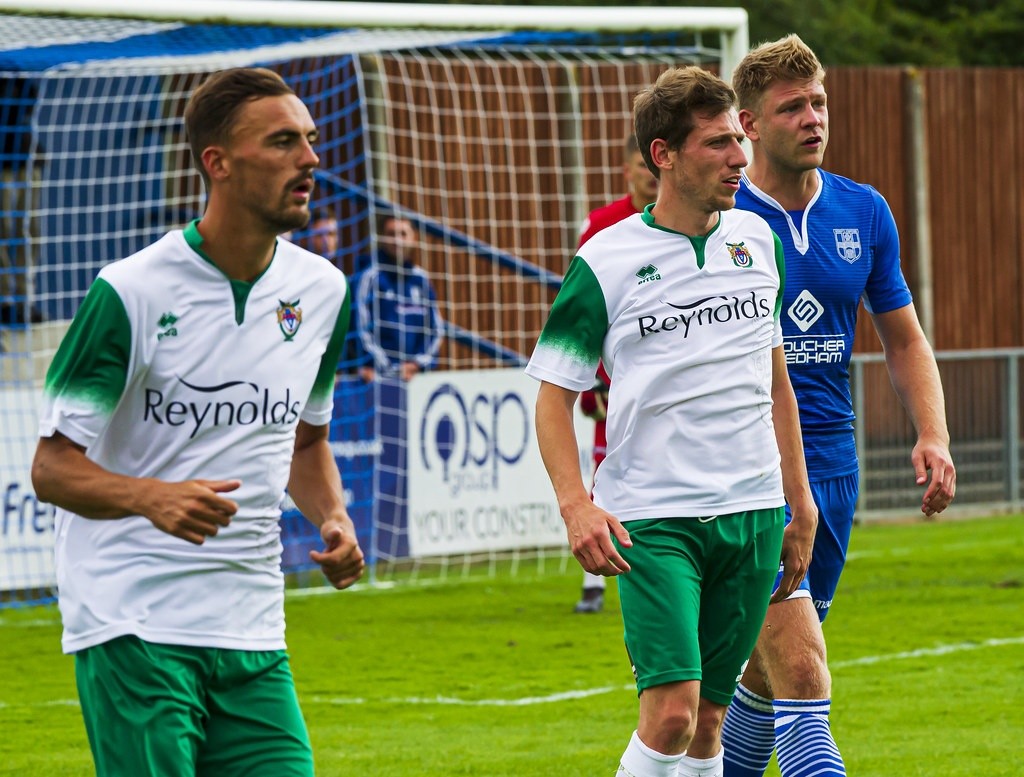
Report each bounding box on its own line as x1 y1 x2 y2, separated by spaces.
353 215 446 382
527 63 820 777
302 206 340 263
26 68 368 777
708 28 959 777
575 132 659 254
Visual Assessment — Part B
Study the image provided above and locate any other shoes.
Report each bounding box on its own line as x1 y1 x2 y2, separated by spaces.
575 587 606 613
